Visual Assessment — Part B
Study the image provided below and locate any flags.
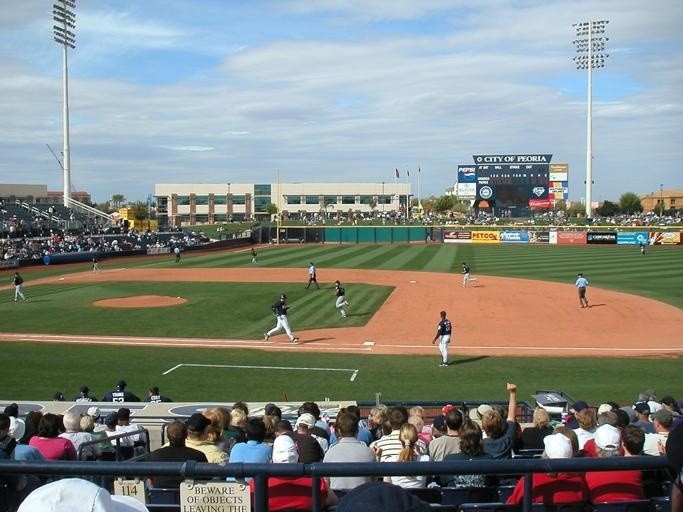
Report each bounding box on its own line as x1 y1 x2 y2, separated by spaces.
395 168 400 179
407 170 410 176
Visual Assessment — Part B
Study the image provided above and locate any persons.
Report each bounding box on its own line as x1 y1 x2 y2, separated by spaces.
480 381 517 487
304 262 319 290
144 400 682 512
575 273 589 308
334 280 349 319
0 198 258 272
263 293 299 343
461 262 478 289
141 385 174 402
1 403 150 495
286 210 683 255
9 272 26 302
432 311 452 367
74 386 99 402
101 379 142 402
53 391 64 401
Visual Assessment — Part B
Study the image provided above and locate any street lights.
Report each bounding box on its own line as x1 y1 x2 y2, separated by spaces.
570 18 611 220
52 0 83 208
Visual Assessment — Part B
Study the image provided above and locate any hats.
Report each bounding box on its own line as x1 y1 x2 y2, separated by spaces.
634 403 650 415
594 424 621 451
478 404 493 415
116 381 126 387
296 413 316 429
272 435 299 464
87 406 100 419
543 433 573 459
572 401 612 414
434 405 455 431
7 416 26 441
81 386 89 393
187 413 211 432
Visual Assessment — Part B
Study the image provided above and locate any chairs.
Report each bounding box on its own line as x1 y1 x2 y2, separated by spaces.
0 200 211 264
0 406 681 512
